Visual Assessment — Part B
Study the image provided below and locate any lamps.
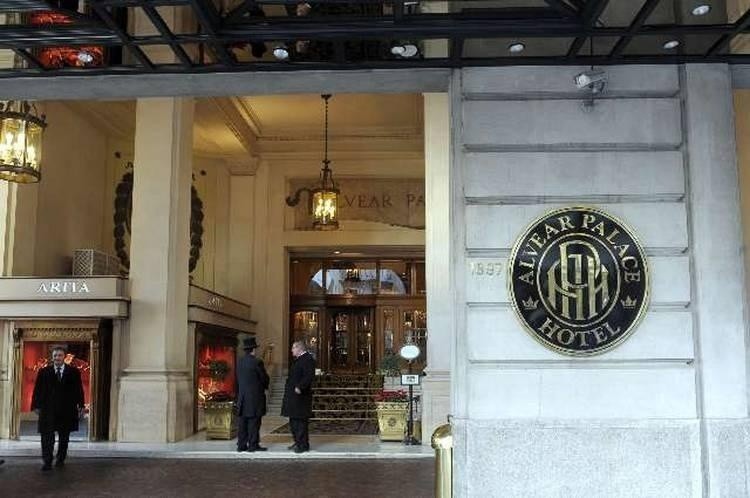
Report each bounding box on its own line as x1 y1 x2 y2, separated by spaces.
307 93 343 232
1 53 47 183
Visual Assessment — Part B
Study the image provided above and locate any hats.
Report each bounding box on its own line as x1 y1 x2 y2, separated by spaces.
241 337 260 350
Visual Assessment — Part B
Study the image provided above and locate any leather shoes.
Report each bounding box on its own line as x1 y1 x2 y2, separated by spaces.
289 444 309 452
56 461 64 467
237 446 268 452
42 464 52 470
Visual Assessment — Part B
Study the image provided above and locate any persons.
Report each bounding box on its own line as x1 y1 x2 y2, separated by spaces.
235 337 270 451
31 346 85 472
280 341 314 454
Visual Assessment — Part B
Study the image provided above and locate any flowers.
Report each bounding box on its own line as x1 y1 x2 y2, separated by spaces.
373 389 407 403
203 390 231 402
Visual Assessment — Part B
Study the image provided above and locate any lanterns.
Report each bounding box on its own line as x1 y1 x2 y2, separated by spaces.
0 110 49 184
312 180 341 232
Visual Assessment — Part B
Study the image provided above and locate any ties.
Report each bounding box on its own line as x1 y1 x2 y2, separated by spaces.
56 367 61 382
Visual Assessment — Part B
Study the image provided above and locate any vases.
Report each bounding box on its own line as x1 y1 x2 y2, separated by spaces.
206 401 238 439
376 399 409 443
